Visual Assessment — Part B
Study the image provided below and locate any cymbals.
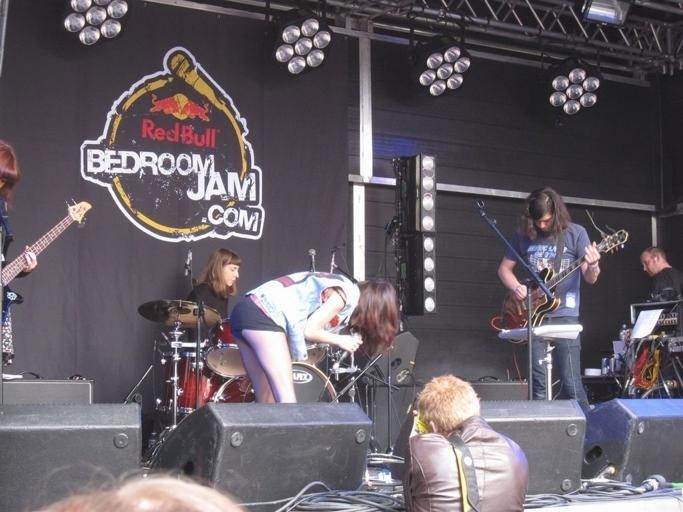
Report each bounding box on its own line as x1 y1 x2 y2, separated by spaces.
138 300 220 328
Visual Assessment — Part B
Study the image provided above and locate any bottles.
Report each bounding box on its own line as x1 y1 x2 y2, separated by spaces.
618 324 627 342
600 353 624 376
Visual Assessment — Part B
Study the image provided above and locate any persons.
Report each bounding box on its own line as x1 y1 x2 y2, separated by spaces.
181 247 241 409
36 461 244 512
0 141 38 366
497 187 601 414
402 374 529 512
640 246 683 303
230 272 401 403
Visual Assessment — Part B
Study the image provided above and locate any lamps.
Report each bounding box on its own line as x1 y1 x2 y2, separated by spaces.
60 0 130 48
544 50 604 122
264 0 335 78
401 17 473 98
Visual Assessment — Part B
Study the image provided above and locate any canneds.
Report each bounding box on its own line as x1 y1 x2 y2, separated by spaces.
601 358 610 375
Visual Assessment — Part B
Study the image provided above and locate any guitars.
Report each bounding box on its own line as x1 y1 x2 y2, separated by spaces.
1 198 91 326
501 230 629 345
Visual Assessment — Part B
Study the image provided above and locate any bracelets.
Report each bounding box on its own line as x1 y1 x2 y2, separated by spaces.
589 261 598 267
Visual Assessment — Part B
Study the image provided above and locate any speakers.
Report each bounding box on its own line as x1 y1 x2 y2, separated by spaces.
582 398 683 487
149 403 373 511
362 330 425 389
480 399 586 494
0 403 143 511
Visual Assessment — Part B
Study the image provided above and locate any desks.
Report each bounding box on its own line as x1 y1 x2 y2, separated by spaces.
581 375 619 404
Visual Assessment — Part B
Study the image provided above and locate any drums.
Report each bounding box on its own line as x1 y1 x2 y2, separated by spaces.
157 343 221 415
206 317 249 377
212 363 338 403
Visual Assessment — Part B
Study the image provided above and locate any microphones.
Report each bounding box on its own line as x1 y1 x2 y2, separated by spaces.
329 250 337 273
309 248 317 272
185 251 193 277
